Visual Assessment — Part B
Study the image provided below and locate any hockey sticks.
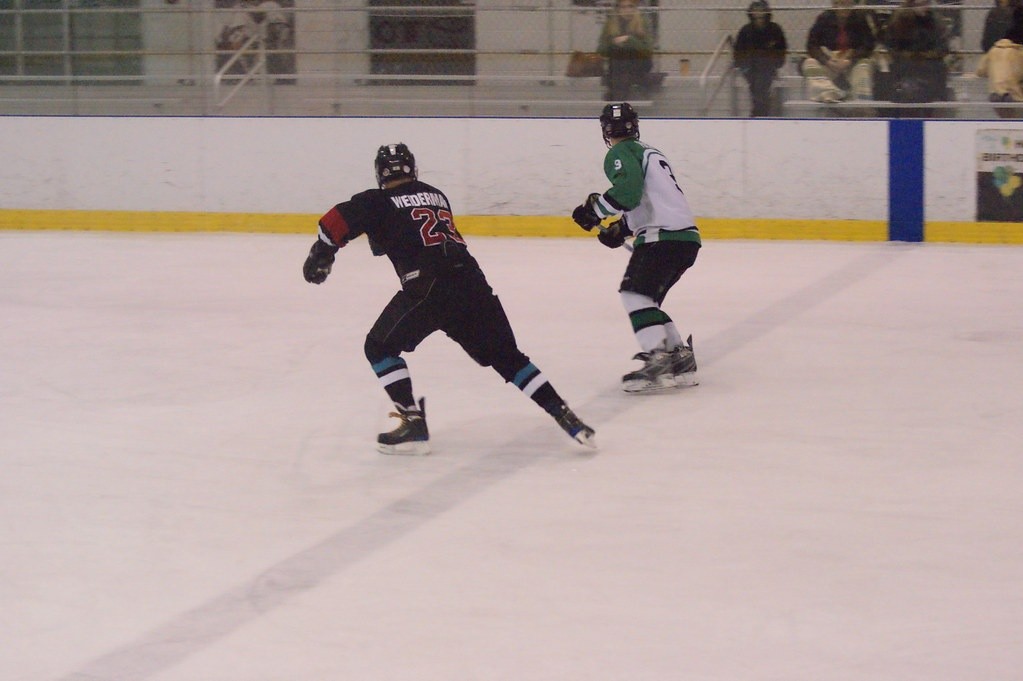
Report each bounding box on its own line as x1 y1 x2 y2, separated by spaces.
594 221 636 255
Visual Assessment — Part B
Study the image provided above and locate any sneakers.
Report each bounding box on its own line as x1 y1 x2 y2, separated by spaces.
672 334 699 386
621 338 676 392
377 396 431 456
556 405 597 450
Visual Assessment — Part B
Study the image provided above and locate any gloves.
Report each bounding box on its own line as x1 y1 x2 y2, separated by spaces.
597 213 634 249
571 193 606 231
302 240 339 285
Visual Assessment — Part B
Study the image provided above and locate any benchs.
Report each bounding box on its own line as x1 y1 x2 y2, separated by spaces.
0 51 990 118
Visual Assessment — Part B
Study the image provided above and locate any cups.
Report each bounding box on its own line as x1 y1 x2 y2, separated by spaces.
680 58 689 76
874 49 891 73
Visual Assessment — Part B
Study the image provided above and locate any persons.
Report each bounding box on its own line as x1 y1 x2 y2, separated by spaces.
803 0 961 118
976 0 1023 118
302 143 597 456
596 0 652 102
574 103 700 391
730 0 787 117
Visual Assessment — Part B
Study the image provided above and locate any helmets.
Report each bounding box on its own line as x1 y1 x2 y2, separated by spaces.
374 143 416 190
599 102 640 149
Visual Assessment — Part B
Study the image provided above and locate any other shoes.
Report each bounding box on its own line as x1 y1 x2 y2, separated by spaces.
990 91 1015 119
